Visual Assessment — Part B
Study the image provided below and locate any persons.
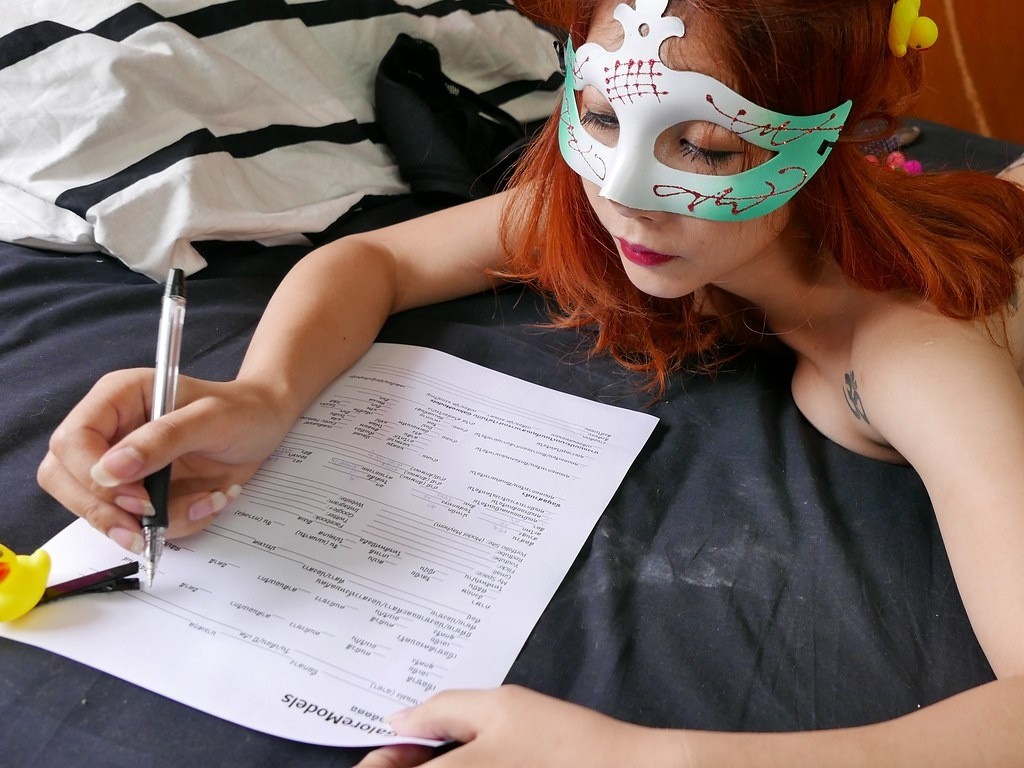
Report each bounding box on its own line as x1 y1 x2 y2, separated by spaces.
35 0 1024 768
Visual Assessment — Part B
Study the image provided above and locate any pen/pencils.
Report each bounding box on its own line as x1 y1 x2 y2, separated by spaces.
139 268 189 587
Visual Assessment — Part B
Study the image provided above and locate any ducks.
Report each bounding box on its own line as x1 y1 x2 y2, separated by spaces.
887 0 938 58
0 542 52 626
